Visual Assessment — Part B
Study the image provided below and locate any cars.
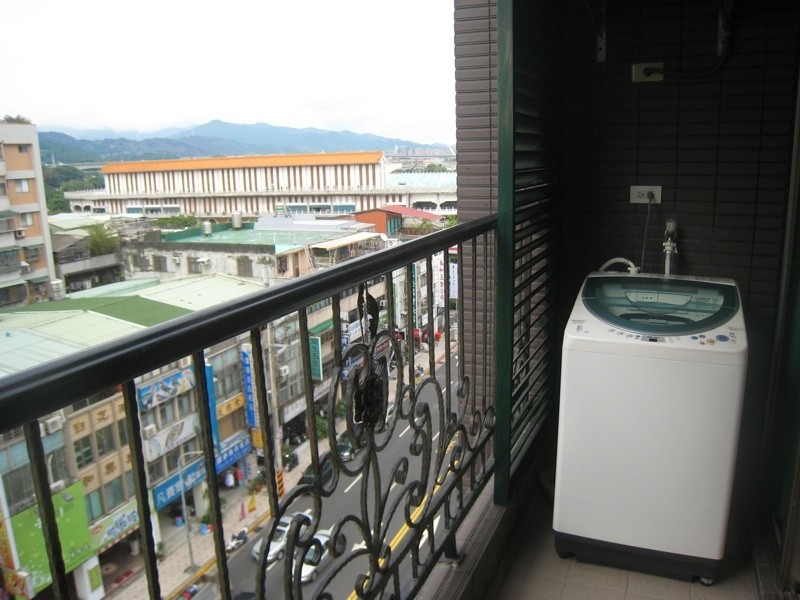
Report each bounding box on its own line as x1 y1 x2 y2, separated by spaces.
294 453 334 496
251 511 313 564
293 529 332 585
335 429 362 462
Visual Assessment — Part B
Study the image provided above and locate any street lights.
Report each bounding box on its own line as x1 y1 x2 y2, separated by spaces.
178 450 205 571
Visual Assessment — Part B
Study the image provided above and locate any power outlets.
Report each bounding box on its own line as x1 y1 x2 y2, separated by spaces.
630 62 665 82
629 185 662 204
596 31 606 64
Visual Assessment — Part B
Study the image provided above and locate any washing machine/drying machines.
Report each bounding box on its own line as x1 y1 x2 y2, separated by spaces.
550 268 750 592
